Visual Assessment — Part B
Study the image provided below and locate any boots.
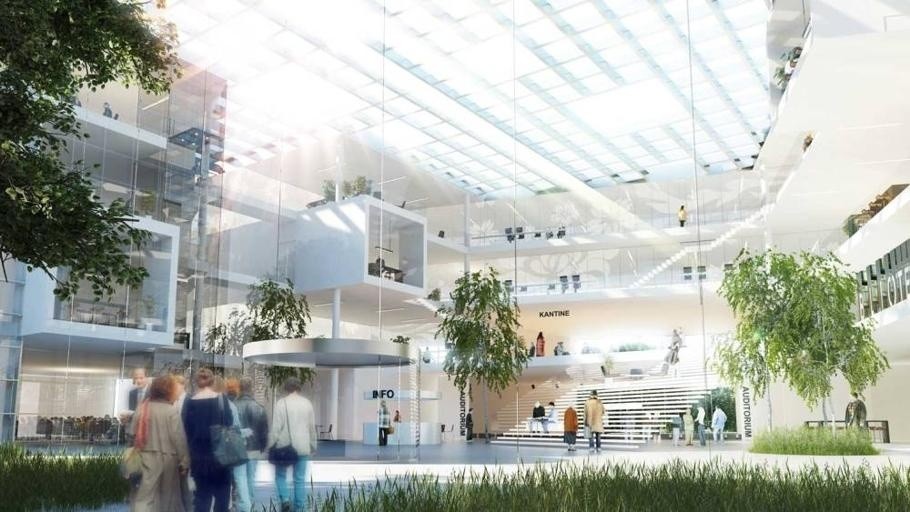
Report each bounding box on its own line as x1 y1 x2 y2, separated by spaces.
568 444 576 452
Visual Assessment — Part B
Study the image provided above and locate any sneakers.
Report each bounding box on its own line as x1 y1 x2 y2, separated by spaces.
589 447 594 451
596 447 602 451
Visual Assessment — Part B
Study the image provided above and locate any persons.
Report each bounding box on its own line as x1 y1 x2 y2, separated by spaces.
845 392 867 427
379 400 391 446
466 408 475 442
393 410 401 422
679 205 686 226
123 367 317 512
525 328 728 454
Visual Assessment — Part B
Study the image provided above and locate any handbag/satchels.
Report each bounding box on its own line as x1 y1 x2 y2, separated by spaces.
209 425 250 468
583 424 593 439
268 445 300 465
387 424 396 435
119 446 146 489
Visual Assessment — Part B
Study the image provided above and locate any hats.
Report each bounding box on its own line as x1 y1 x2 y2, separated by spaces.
589 389 598 396
